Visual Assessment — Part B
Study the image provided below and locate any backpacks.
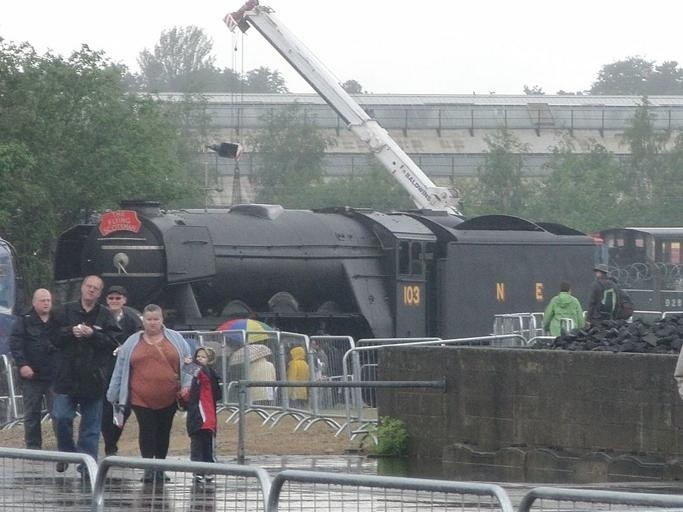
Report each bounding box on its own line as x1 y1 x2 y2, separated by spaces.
610 289 634 321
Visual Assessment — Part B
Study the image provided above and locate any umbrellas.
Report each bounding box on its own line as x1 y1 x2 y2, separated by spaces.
213 318 281 348
226 342 273 367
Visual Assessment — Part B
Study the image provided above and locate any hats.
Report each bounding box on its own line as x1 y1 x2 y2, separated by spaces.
108 285 128 296
591 264 609 273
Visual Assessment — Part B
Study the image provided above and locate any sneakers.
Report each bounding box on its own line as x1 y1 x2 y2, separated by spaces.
138 471 215 483
56 461 69 472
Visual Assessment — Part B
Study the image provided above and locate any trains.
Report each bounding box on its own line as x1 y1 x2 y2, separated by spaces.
0 201 681 407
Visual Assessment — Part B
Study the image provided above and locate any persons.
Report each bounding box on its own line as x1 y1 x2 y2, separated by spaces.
310 337 328 410
318 333 340 406
47 274 123 480
93 284 143 458
541 281 586 338
7 289 68 452
584 263 622 321
105 302 194 484
286 346 308 409
246 358 276 406
179 343 221 484
605 269 633 318
